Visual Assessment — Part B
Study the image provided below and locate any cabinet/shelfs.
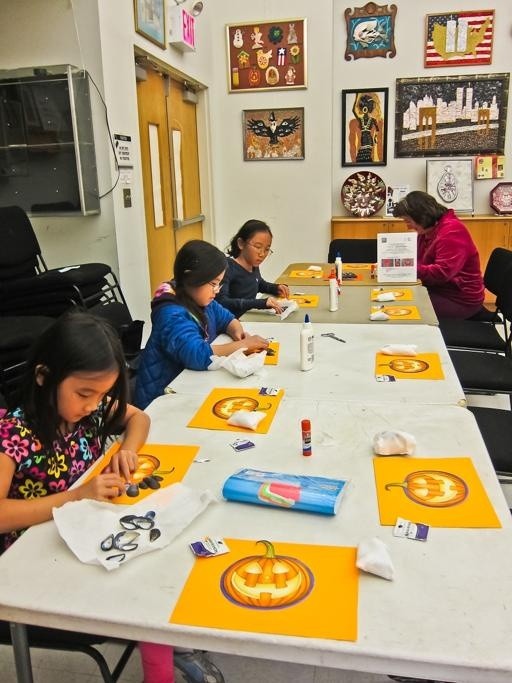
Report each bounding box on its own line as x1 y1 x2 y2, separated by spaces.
328 214 511 305
1 62 102 221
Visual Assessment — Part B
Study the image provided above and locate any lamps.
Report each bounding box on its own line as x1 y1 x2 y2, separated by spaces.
175 0 205 17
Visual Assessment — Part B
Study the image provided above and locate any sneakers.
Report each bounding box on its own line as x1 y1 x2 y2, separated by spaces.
173 648 226 683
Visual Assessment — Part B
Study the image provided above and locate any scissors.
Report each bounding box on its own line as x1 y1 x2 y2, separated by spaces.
321 332 347 344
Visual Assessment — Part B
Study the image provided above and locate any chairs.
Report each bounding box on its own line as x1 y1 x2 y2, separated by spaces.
428 246 510 323
1 199 143 365
326 238 380 264
435 264 512 354
0 355 141 682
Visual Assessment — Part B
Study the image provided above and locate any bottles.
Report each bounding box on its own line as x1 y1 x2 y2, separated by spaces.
328 268 338 312
301 419 312 456
335 251 343 286
300 314 317 371
371 265 374 279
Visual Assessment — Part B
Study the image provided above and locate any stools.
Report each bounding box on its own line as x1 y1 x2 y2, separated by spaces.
447 346 510 393
465 405 511 485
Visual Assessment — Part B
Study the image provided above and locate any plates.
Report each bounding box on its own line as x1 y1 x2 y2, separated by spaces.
340 170 386 216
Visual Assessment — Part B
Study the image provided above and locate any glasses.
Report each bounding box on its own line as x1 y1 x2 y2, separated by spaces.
208 282 224 291
248 243 273 257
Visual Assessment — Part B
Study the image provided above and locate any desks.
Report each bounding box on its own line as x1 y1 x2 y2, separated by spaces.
0 387 510 681
160 318 472 407
273 261 424 285
238 285 440 326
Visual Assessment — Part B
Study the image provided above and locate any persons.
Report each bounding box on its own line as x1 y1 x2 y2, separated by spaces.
0 313 152 552
131 239 269 410
213 219 291 315
393 190 485 321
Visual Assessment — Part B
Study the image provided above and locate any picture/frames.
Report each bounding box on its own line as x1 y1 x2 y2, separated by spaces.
338 86 390 166
425 157 477 219
131 0 169 51
239 105 306 163
339 1 397 61
222 13 307 94
394 68 510 159
420 7 497 68
